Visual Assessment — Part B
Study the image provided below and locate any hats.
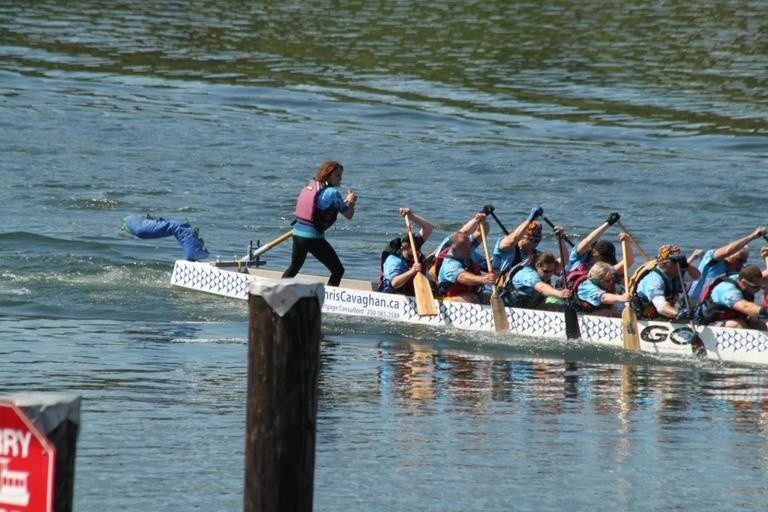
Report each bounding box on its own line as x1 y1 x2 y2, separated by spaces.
594 240 617 265
523 220 542 242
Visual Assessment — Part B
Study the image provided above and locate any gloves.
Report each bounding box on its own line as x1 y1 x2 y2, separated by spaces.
527 206 543 222
605 213 620 226
671 255 688 268
675 308 694 320
482 205 495 216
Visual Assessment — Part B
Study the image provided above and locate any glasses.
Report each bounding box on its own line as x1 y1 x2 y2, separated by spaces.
745 280 760 288
542 268 552 273
736 257 747 264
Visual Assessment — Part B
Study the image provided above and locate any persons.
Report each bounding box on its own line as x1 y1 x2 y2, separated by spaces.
379 208 443 301
280 160 356 286
429 204 768 333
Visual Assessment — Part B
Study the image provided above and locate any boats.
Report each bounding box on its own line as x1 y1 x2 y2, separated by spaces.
170 259 768 364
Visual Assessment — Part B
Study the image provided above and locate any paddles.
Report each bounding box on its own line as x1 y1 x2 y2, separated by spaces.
555 231 580 340
622 237 639 351
478 221 510 333
676 260 707 359
405 214 437 316
